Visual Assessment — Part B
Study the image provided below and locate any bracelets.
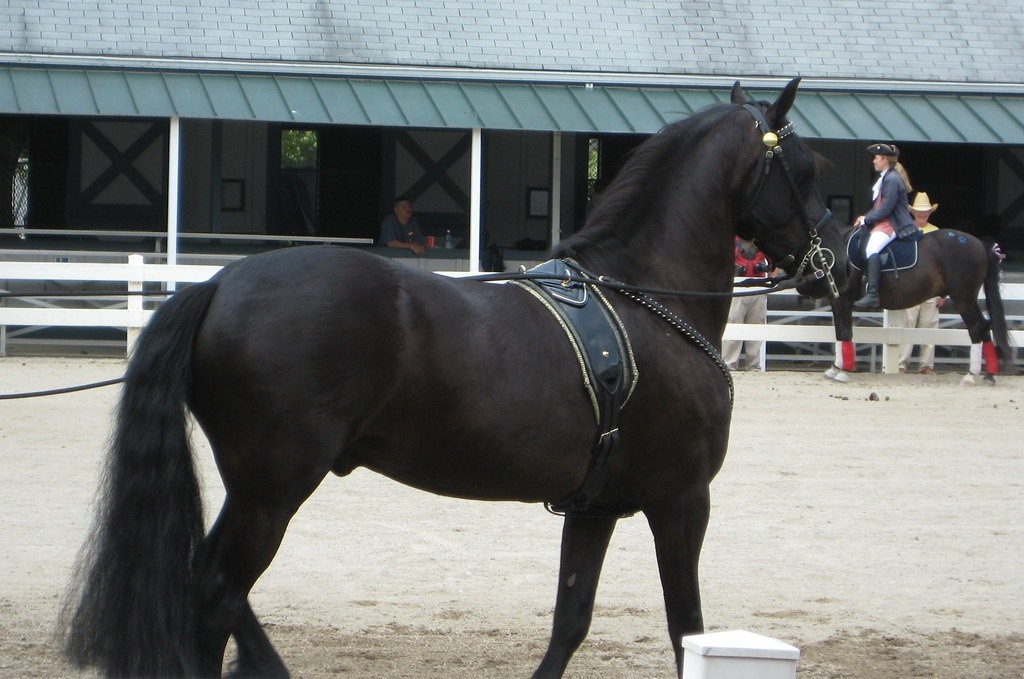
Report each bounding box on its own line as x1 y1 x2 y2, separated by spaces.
408 242 412 250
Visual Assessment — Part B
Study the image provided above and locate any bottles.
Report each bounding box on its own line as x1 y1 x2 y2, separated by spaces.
409 232 416 242
446 230 452 248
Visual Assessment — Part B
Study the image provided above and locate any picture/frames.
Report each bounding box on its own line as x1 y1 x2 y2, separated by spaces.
827 194 855 226
221 178 248 214
524 186 551 220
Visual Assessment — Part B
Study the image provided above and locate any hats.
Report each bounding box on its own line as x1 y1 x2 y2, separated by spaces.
907 191 938 213
867 144 899 156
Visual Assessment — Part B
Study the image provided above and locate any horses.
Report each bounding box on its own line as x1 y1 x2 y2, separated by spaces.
58 76 853 679
824 216 1014 386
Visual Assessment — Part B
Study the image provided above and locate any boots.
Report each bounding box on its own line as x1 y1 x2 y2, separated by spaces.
852 253 881 308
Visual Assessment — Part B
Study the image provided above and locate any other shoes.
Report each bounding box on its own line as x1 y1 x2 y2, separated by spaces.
921 368 935 374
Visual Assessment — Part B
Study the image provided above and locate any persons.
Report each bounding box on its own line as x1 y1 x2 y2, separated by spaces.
378 197 426 255
852 144 916 310
898 192 945 374
721 234 778 371
